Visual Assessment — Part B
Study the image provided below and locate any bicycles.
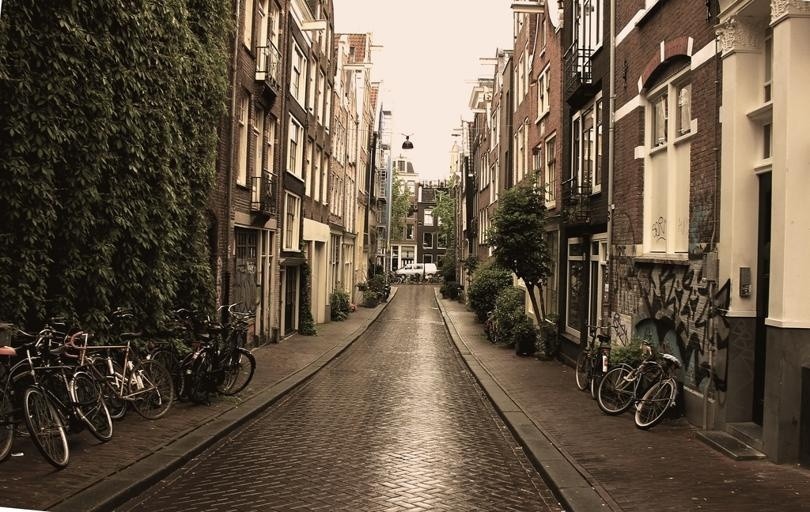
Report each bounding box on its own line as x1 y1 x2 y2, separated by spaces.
570 323 621 401
598 338 682 427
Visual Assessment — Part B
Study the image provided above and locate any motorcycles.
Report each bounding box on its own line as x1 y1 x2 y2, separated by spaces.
387 270 435 284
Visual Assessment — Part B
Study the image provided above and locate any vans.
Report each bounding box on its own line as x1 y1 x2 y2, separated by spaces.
393 263 437 280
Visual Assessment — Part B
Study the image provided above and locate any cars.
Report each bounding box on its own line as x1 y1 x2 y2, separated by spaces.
436 270 444 282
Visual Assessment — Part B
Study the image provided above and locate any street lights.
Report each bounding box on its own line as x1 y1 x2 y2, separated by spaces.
366 128 417 288
